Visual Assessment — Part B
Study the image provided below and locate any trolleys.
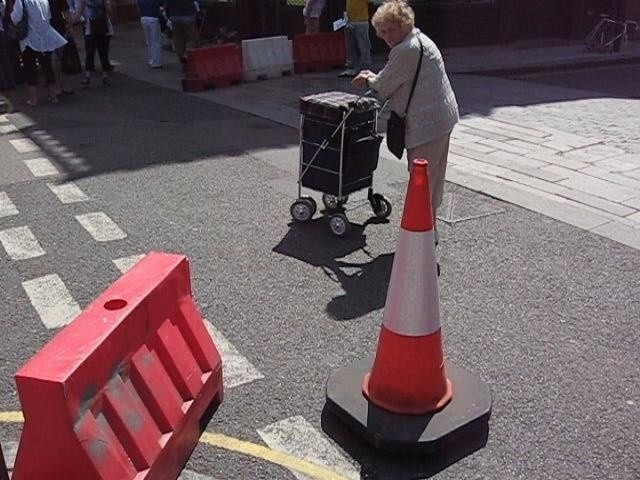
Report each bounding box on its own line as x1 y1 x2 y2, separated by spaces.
291 83 393 238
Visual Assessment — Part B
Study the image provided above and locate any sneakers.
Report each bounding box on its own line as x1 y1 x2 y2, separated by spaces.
338 68 357 78
80 77 91 85
102 76 112 85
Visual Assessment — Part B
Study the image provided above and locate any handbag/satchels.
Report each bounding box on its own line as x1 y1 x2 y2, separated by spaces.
5 0 29 42
63 37 81 73
386 109 406 160
90 15 109 34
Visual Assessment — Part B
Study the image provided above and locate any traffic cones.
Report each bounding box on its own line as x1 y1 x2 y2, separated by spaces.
323 158 497 454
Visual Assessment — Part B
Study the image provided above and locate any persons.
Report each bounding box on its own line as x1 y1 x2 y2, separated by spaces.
302 0 326 32
350 0 459 250
0 0 115 106
138 0 197 69
336 0 372 77
